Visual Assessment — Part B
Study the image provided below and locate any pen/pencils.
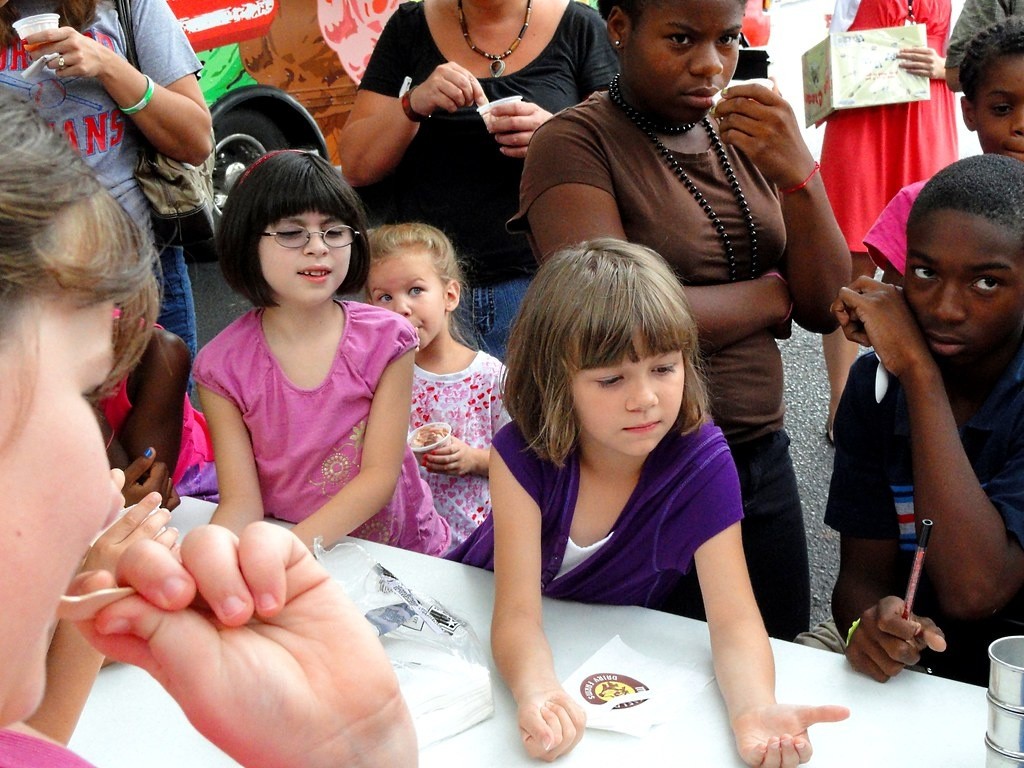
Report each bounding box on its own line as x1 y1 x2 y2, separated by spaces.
900 518 934 620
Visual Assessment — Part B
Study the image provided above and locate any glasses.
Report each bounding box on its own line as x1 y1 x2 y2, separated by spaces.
262 225 361 248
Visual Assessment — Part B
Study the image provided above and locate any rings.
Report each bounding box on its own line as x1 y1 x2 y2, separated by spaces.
59 55 64 70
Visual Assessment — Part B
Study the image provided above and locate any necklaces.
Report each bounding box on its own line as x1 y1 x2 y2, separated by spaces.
457 0 531 79
609 72 757 283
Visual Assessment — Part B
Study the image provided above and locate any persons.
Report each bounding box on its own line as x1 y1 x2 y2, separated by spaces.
0 0 1024 768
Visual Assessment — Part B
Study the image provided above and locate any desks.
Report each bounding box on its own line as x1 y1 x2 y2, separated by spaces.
70 496 990 768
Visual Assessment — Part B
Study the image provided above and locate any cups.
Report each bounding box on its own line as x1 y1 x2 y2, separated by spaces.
477 96 524 134
12 13 59 51
408 422 451 470
712 78 773 107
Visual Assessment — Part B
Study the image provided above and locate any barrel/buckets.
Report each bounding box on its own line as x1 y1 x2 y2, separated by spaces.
983 636 1024 768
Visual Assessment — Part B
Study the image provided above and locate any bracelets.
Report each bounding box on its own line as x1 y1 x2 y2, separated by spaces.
760 272 793 326
845 617 860 648
402 86 431 123
118 73 155 115
106 429 114 451
779 162 819 193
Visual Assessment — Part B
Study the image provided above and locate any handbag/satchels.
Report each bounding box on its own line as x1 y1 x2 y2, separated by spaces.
113 0 216 219
